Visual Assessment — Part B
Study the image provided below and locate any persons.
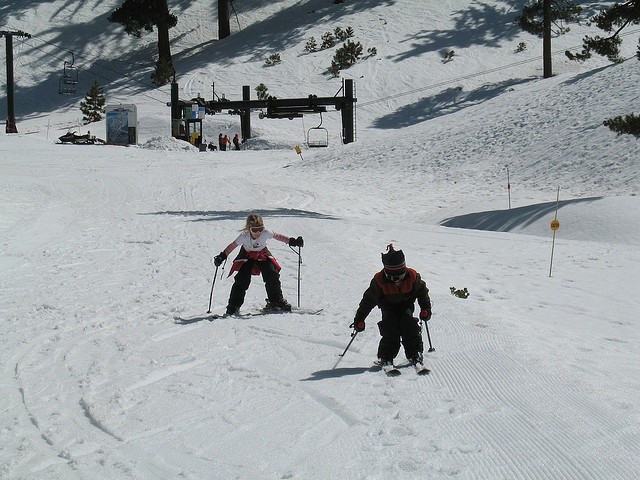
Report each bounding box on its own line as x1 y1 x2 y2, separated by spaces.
219 133 224 151
233 133 241 151
355 241 432 366
221 135 231 151
212 214 304 314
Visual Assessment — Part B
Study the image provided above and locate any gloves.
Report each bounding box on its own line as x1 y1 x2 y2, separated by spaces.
289 236 303 247
214 252 227 266
354 318 365 332
419 308 431 321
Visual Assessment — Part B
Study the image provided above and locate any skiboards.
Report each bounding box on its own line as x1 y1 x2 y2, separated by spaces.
212 307 323 318
374 360 431 375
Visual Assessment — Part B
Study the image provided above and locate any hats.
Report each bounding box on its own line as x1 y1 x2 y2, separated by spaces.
381 243 406 276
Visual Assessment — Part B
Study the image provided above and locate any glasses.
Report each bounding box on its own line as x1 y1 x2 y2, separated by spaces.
249 225 265 235
384 269 406 282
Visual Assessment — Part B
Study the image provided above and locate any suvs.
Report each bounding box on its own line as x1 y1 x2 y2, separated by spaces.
54 130 91 145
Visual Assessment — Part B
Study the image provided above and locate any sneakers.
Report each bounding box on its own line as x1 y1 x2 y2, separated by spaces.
268 295 291 311
381 357 396 373
411 351 424 367
223 305 239 318
